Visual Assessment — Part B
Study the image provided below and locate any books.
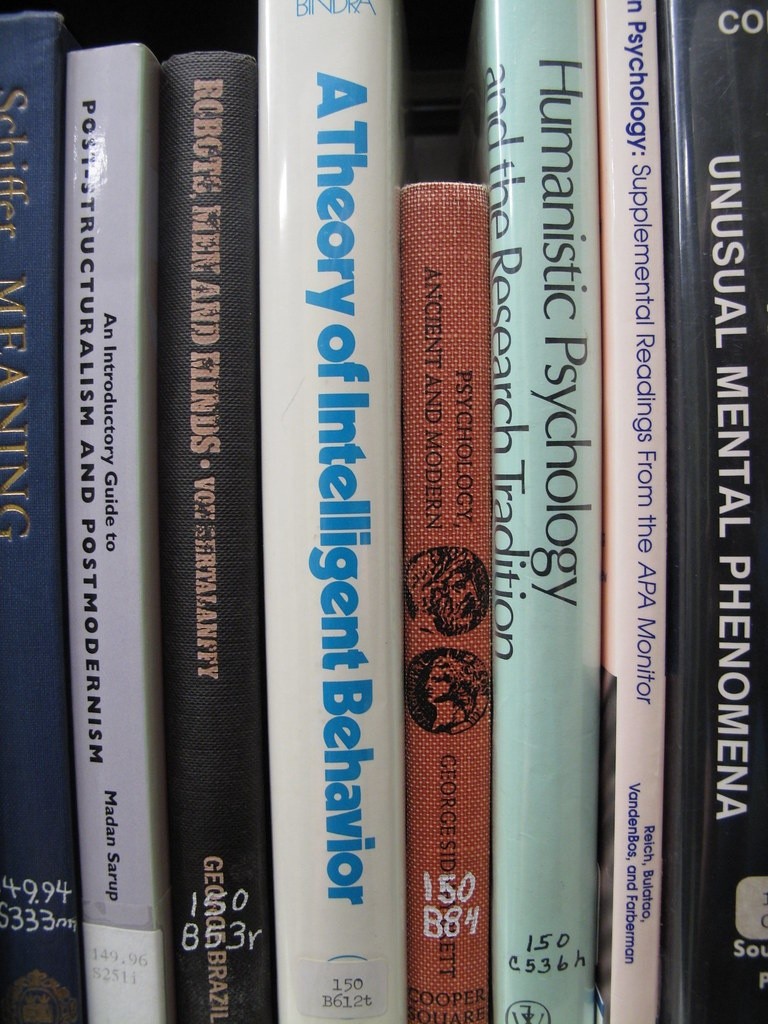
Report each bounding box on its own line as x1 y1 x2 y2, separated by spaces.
0 1 768 1024
